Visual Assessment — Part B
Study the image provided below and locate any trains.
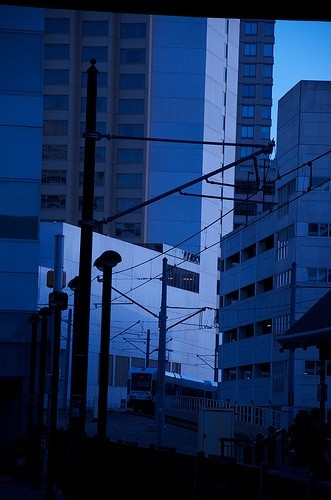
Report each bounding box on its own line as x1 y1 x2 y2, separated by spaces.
126 365 219 420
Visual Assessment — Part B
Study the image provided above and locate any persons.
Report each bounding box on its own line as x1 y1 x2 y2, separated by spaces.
281 406 330 480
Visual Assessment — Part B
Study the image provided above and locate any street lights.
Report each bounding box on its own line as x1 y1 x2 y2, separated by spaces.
92 250 122 443
27 275 79 461
